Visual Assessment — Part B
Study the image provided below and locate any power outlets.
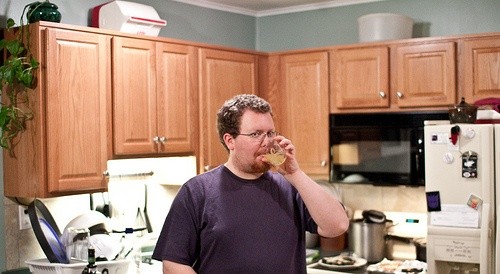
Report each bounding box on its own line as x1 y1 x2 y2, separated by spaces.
19 205 32 230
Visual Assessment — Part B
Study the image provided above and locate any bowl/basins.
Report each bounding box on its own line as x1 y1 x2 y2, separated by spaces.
449 98 478 123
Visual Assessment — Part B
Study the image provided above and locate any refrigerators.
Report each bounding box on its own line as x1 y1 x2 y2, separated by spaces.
422 123 500 274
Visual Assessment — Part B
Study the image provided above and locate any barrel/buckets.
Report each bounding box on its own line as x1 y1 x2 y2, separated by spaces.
349 219 387 263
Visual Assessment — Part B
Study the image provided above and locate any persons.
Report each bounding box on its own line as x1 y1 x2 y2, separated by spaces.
153 94 349 274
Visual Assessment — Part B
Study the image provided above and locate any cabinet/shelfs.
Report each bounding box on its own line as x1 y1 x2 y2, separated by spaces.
0 21 500 198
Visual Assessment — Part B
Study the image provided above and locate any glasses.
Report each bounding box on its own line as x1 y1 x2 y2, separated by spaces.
230 130 276 139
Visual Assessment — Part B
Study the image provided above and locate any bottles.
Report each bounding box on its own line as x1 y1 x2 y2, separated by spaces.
81 248 97 274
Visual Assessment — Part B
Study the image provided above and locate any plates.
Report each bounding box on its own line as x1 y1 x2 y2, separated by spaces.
319 256 367 269
306 248 323 267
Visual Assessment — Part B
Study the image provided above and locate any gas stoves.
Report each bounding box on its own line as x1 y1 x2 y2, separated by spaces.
306 210 427 274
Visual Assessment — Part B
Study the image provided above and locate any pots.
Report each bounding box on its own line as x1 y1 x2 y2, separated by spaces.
385 235 427 262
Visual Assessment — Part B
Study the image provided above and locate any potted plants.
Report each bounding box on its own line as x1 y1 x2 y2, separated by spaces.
0 0 61 148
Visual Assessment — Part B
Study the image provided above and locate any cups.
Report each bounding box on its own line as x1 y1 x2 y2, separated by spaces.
320 232 345 257
257 138 289 167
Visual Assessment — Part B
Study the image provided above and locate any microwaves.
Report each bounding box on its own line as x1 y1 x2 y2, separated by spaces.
328 112 447 187
